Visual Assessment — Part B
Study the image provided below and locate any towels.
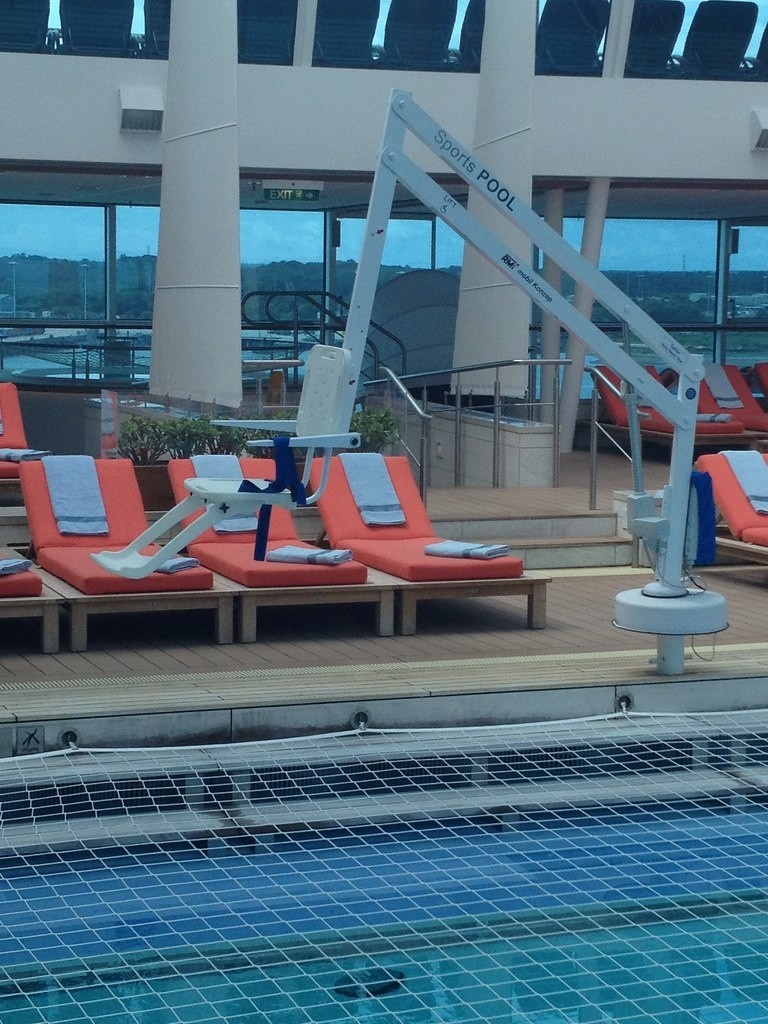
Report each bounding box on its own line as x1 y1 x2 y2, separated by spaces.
717 450 768 514
337 453 407 527
0 417 3 435
265 544 353 566
0 558 33 576
0 448 51 462
424 539 510 560
102 389 115 436
41 455 109 536
152 557 200 575
702 362 744 409
189 455 259 535
636 395 653 408
696 413 734 423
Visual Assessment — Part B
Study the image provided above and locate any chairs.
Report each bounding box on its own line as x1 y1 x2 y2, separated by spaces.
0 383 551 654
695 451 768 565
579 362 768 455
